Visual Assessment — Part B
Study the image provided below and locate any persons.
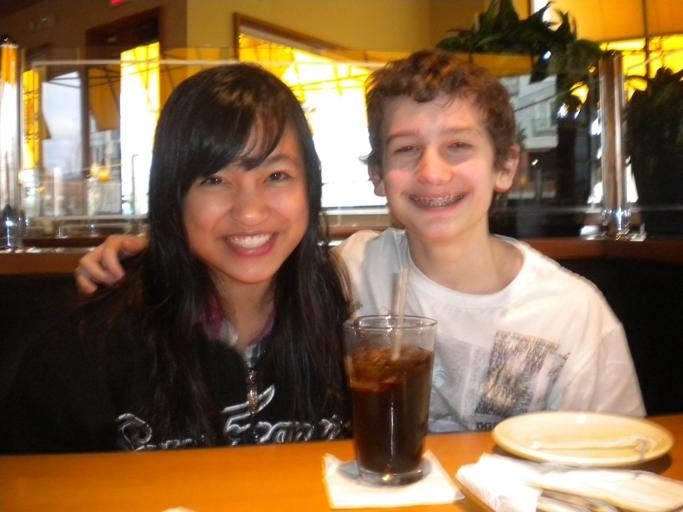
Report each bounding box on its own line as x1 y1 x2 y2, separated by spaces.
1 63 364 455
74 51 645 433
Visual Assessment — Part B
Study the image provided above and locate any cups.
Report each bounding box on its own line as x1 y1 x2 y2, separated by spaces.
344 316 438 487
6 216 29 250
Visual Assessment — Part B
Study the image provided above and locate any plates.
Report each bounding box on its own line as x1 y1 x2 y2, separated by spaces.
492 409 674 468
